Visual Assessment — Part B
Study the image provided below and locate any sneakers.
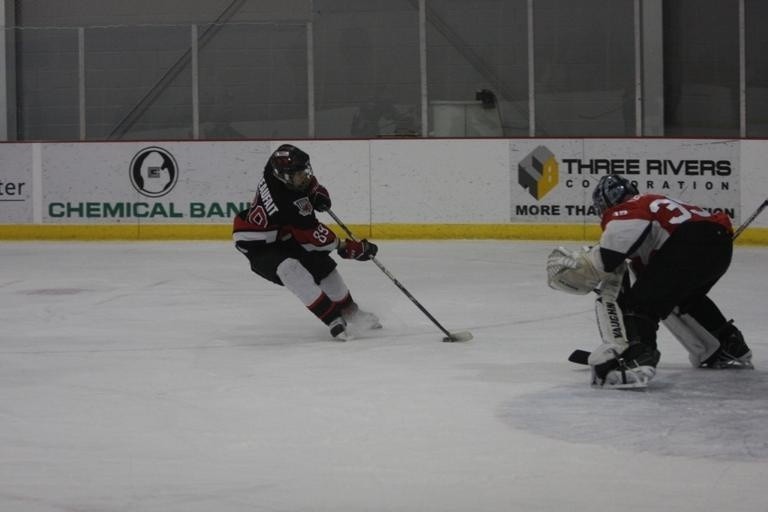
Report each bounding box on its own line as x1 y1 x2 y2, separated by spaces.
327 309 383 343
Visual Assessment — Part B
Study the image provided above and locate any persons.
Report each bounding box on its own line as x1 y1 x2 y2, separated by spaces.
231 144 378 338
542 174 752 384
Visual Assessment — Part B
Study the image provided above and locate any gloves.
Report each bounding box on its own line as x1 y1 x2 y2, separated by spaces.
307 184 333 212
337 237 379 263
544 246 610 296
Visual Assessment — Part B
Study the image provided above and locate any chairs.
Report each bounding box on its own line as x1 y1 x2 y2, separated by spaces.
431 99 505 137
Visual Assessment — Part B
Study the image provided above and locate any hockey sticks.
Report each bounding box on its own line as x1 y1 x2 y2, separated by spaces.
320 203 474 343
569 198 768 365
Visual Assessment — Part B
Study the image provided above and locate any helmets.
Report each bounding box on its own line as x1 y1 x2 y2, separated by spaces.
590 172 640 215
263 143 315 194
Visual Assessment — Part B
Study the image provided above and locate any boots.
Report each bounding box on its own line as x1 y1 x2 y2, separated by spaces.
702 332 750 361
594 344 661 378
